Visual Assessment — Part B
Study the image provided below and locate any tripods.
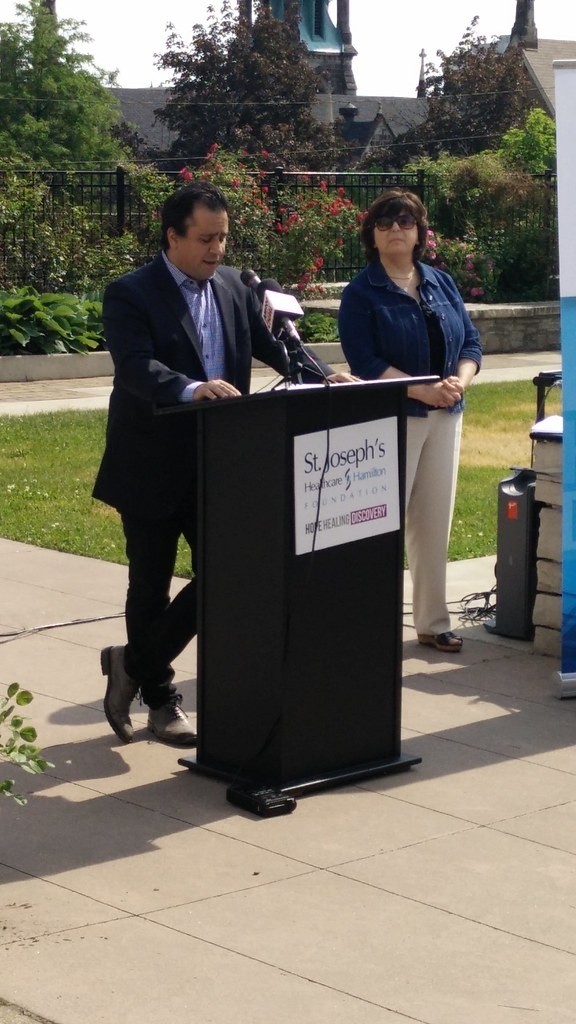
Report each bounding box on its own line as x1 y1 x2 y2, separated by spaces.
271 335 338 392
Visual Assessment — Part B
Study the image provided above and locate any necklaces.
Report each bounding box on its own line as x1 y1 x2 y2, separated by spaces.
388 276 411 280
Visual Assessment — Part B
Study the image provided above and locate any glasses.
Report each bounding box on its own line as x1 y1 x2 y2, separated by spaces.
371 215 417 231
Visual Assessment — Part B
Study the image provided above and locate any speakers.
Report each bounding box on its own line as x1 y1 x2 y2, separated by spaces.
495 468 547 641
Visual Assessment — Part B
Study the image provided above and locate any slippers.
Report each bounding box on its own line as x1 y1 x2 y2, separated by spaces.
418 632 463 652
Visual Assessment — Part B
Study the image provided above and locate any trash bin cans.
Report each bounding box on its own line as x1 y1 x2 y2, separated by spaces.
496 467 538 641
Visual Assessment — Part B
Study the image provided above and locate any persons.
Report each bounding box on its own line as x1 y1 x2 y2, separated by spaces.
336 191 482 651
94 182 364 748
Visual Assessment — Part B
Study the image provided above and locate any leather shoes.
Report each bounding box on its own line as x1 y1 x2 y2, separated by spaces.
101 645 141 743
148 697 197 744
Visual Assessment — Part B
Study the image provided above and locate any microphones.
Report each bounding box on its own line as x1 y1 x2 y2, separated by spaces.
240 269 305 349
